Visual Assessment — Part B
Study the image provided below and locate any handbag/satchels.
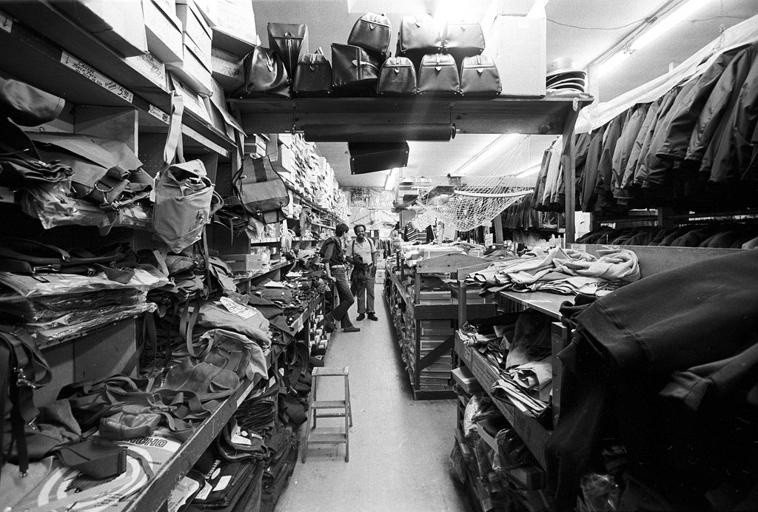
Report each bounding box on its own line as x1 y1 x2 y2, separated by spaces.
237 14 503 98
229 150 291 223
161 297 273 414
1 124 163 480
149 157 212 253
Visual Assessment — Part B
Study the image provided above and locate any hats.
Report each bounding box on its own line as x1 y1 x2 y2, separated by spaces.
546 57 586 91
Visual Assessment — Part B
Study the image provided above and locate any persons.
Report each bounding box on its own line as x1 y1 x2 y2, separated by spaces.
323 223 360 333
346 224 378 322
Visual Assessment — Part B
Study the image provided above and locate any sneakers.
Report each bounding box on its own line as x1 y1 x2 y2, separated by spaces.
325 312 335 330
368 313 377 321
344 327 360 332
356 313 365 321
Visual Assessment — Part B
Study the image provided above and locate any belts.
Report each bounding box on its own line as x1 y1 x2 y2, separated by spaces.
332 264 344 269
